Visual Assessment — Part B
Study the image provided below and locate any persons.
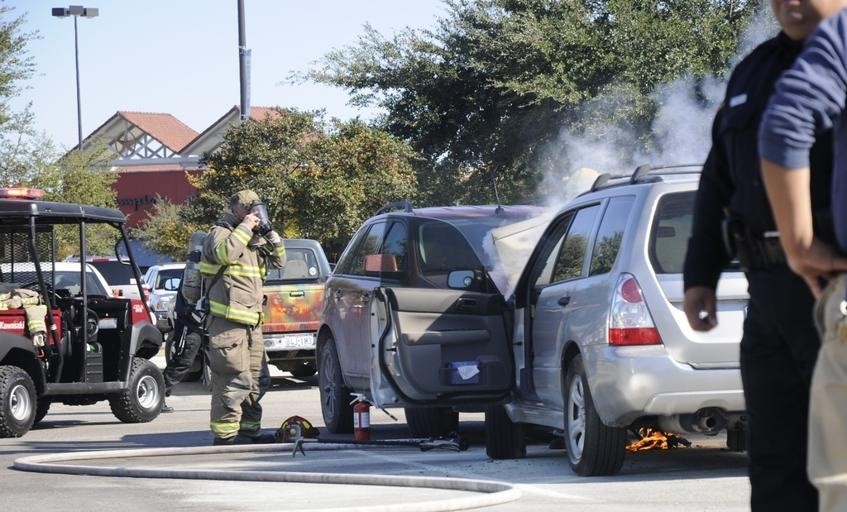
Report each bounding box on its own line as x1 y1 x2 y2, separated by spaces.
160 268 211 413
683 0 847 512
757 6 847 512
198 189 287 446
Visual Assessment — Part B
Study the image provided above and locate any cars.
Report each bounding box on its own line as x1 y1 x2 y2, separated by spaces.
0 263 113 298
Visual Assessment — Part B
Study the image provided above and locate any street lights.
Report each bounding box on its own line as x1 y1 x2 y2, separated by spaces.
51 2 98 151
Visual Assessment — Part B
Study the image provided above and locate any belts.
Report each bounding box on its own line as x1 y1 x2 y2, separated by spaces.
724 230 794 276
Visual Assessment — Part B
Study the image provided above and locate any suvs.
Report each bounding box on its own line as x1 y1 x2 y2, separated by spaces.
72 254 152 327
143 257 189 342
259 236 333 381
313 166 557 439
478 164 750 477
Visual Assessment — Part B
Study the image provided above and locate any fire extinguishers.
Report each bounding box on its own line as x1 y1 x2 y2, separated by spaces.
350 393 377 442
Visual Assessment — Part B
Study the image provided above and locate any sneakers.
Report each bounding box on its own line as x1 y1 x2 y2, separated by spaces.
213 433 252 446
162 401 174 413
240 432 275 444
